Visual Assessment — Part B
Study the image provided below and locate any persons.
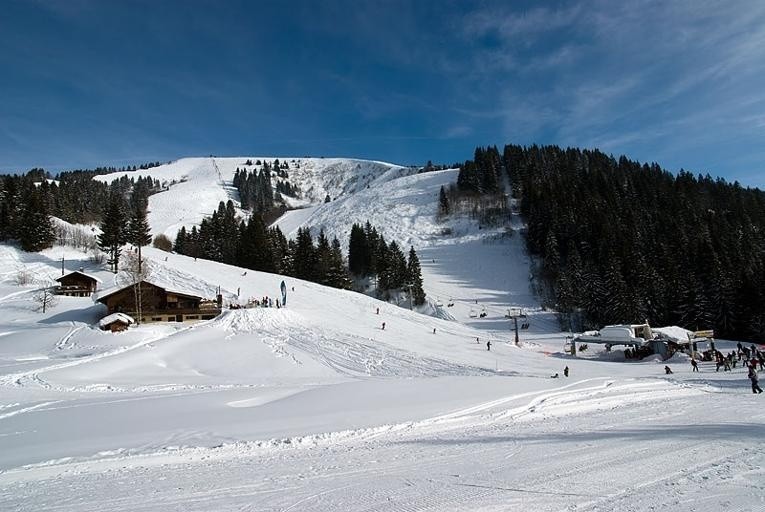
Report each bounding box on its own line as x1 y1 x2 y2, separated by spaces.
580 344 588 351
522 323 530 328
563 365 570 377
551 374 559 378
664 341 765 394
292 286 294 291
381 322 386 330
243 272 247 276
487 341 492 350
624 344 655 360
377 307 380 314
165 257 169 261
194 256 197 261
433 328 437 333
254 295 282 308
477 337 480 344
